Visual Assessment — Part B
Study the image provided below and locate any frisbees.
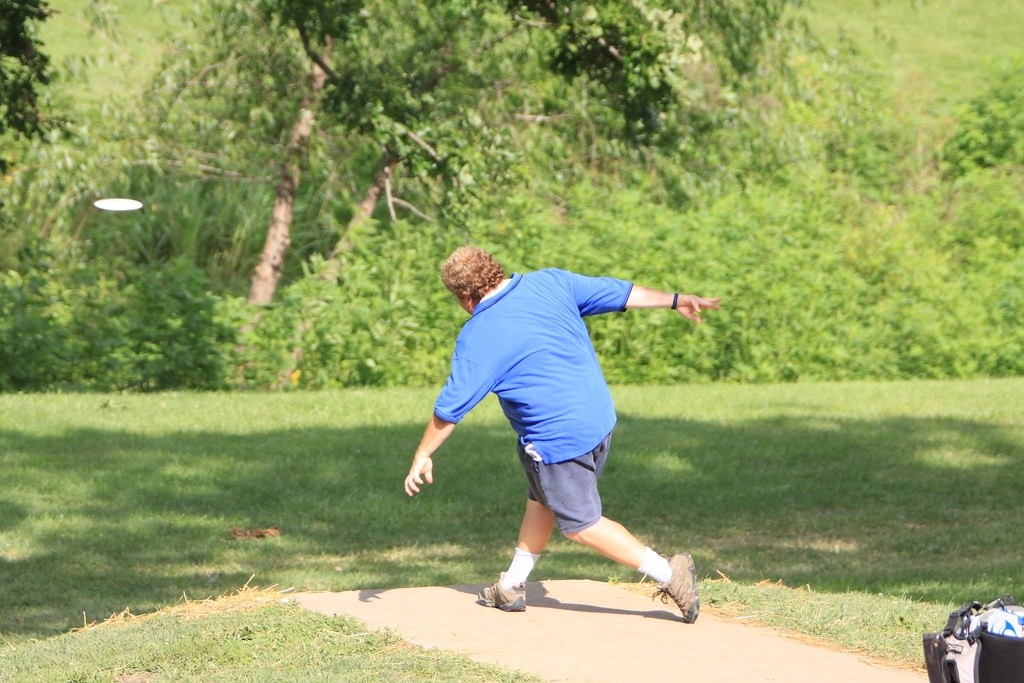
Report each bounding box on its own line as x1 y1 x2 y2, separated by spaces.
94 198 143 211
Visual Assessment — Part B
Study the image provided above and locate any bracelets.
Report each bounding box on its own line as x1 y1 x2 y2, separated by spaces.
671 293 679 310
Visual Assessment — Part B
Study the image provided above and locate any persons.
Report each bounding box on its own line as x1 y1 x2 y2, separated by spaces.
405 244 725 624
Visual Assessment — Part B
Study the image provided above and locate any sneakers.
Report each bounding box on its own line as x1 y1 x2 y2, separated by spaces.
479 572 526 610
651 554 700 623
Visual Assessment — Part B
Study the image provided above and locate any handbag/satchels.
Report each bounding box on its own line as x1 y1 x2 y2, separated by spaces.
924 600 1024 682
